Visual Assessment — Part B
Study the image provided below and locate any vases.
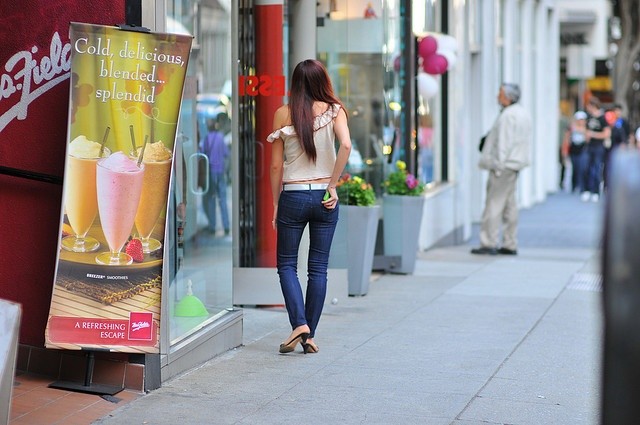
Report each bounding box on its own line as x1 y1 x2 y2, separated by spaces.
382 193 425 275
329 205 379 296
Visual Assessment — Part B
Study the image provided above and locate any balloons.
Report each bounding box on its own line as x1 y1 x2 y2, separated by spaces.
418 36 436 59
419 55 448 74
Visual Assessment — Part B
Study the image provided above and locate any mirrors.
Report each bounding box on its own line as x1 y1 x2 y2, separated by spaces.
158 1 234 346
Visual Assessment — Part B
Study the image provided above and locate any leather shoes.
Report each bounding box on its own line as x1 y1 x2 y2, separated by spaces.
497 248 516 255
472 247 497 254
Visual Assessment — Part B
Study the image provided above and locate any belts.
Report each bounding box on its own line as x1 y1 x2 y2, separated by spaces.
282 183 329 191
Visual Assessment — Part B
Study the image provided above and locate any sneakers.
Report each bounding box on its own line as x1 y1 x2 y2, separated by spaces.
591 193 599 202
581 191 590 201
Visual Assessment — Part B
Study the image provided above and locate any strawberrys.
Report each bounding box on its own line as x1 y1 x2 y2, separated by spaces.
126 238 144 263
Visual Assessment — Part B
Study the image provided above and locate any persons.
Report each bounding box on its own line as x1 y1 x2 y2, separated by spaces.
268 60 351 354
472 83 528 254
201 119 229 236
561 97 630 202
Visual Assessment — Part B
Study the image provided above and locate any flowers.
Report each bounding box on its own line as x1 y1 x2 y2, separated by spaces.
334 173 376 206
380 161 425 195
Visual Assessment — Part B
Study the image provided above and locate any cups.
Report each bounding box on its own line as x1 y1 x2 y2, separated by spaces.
131 141 172 255
61 133 110 255
97 157 144 267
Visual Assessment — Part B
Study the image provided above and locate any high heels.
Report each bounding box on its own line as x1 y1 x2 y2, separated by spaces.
300 338 319 354
279 324 310 353
